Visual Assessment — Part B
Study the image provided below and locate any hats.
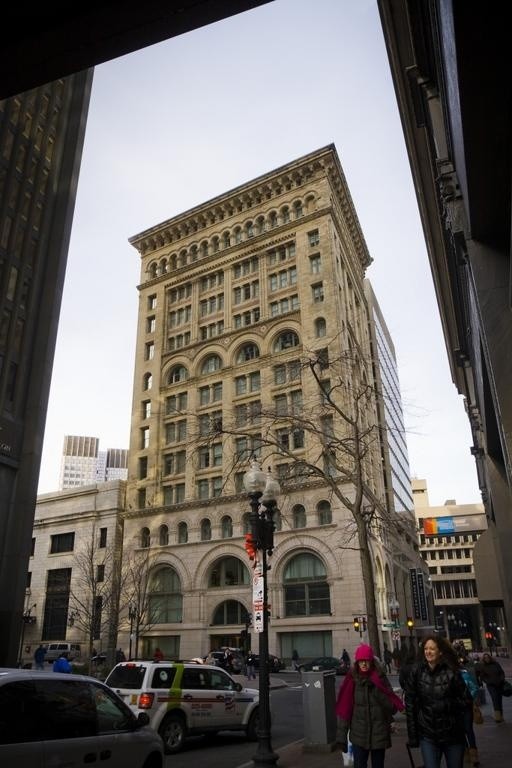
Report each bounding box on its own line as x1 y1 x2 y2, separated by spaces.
355 643 374 660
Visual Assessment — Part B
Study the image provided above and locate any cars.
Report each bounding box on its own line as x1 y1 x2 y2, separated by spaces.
295 657 347 675
1 667 165 766
251 655 286 674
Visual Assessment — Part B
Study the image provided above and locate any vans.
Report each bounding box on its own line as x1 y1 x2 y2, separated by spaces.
43 643 81 663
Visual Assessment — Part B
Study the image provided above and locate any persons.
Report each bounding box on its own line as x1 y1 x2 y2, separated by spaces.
291 650 299 671
221 647 232 672
206 652 216 666
269 656 282 673
34 646 47 670
92 647 97 657
245 650 256 681
54 651 73 674
117 648 126 664
333 637 505 768
153 648 164 661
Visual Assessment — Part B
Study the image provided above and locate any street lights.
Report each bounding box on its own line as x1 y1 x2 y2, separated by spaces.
389 602 401 668
241 455 282 754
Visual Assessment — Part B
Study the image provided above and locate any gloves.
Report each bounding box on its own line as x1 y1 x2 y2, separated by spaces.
337 740 348 754
362 678 376 692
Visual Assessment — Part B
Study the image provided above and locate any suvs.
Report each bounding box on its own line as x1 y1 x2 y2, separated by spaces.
99 659 274 753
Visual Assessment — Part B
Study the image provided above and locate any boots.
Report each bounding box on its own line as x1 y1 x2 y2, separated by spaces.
494 709 504 722
464 746 480 766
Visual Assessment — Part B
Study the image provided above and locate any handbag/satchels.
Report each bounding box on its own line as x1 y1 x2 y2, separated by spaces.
473 704 484 725
503 681 512 697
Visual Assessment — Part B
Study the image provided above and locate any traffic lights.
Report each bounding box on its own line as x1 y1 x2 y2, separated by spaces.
353 618 359 632
407 617 413 630
